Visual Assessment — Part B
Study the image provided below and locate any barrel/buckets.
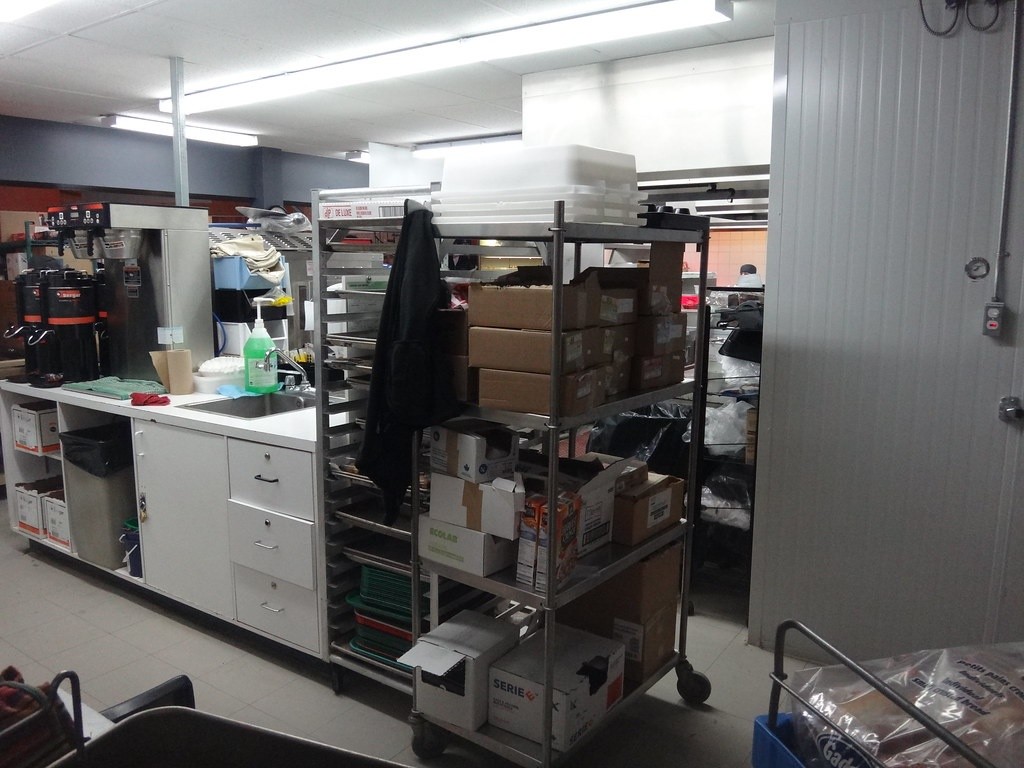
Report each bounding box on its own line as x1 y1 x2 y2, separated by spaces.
118 518 142 578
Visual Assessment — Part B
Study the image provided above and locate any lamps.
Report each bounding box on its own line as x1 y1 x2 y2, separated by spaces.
346 151 370 164
159 0 734 115
101 116 258 147
410 134 522 158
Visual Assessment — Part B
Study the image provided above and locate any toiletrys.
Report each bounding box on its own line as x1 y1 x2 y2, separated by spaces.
242 296 278 393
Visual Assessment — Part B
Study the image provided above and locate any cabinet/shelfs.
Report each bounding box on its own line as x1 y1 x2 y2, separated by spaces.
310 188 711 768
0 391 362 665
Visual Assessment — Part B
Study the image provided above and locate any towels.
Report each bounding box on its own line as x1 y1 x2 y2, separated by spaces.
130 392 171 406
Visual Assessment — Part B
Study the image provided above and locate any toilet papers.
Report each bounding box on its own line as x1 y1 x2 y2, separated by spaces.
148 348 194 395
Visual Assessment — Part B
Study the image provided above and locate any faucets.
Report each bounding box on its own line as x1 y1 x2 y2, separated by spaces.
264 346 316 397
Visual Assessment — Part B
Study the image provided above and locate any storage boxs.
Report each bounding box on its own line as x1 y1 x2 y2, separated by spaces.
12 402 60 457
44 491 70 550
212 255 290 356
14 475 64 540
429 144 651 224
350 419 685 754
467 243 688 417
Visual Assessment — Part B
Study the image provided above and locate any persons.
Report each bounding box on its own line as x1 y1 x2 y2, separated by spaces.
740 264 765 288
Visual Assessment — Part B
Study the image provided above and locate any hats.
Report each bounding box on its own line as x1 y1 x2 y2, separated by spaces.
740 264 756 275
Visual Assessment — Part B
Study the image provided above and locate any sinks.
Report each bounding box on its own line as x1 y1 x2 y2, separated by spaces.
173 390 348 421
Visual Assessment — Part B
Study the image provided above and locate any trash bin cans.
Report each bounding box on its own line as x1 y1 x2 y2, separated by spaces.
619 403 693 481
60 424 137 571
